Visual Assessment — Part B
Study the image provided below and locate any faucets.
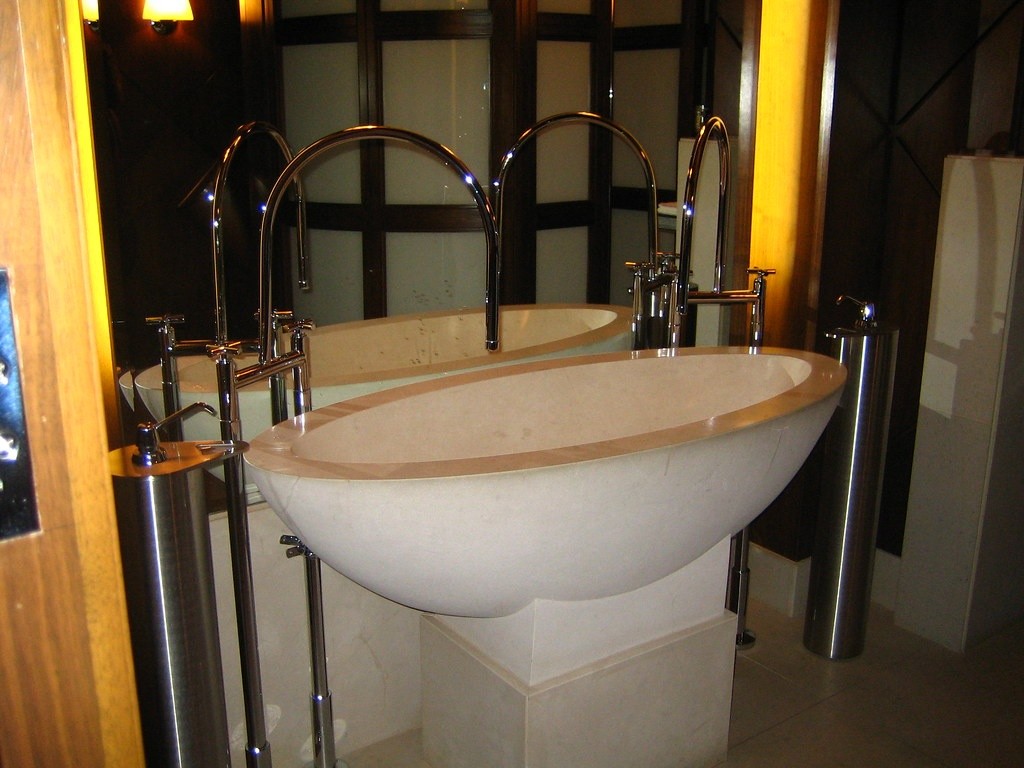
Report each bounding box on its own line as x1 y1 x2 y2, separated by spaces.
213 117 311 346
494 111 658 277
674 116 730 317
259 126 501 363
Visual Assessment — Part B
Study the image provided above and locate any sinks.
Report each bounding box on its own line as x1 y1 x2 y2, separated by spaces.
241 346 849 619
134 303 634 484
118 370 134 411
